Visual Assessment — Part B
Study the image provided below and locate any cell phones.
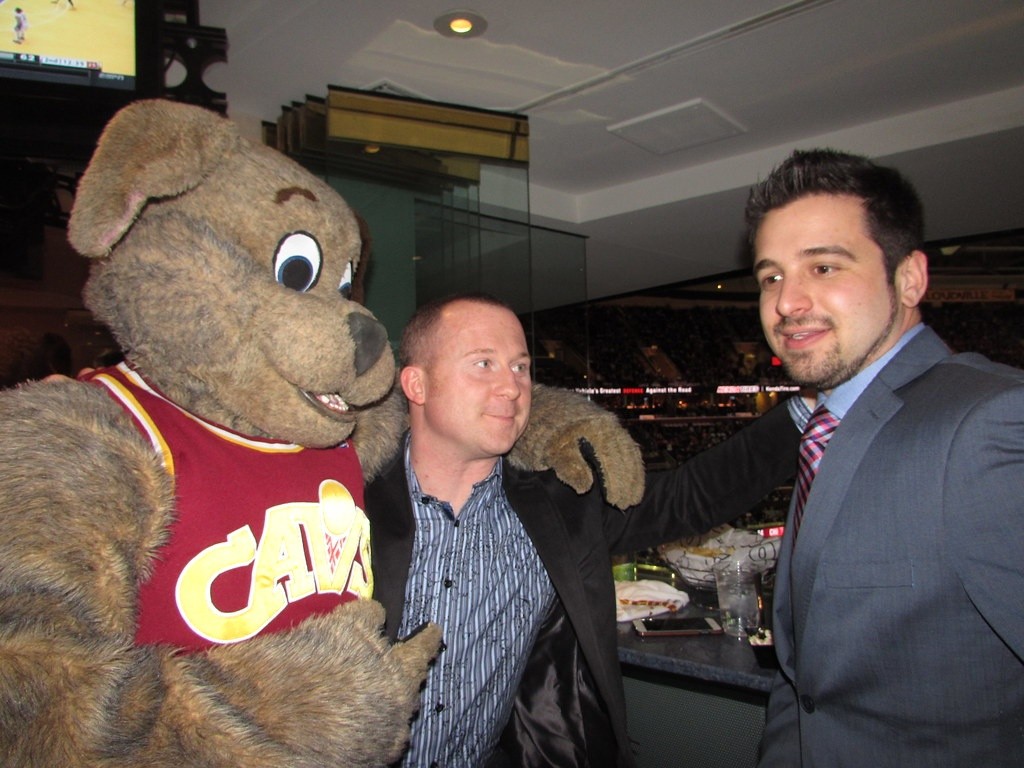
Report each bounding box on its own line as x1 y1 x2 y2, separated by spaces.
633 617 724 636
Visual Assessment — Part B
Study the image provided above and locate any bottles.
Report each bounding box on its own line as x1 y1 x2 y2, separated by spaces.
609 550 637 582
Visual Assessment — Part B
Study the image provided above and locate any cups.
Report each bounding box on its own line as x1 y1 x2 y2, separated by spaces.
712 561 760 638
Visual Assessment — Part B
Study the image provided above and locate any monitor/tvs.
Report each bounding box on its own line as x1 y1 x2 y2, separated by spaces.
0 0 137 92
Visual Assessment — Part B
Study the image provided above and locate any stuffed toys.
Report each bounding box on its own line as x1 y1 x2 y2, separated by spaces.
1 100 644 768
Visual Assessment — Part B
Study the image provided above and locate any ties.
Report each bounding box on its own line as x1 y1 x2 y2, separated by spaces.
793 403 841 549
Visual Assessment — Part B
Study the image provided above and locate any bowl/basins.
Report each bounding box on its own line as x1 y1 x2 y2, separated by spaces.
754 644 780 668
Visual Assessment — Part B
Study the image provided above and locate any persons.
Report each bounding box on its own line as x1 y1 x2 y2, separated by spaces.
740 149 1023 768
0 0 136 44
366 292 819 768
